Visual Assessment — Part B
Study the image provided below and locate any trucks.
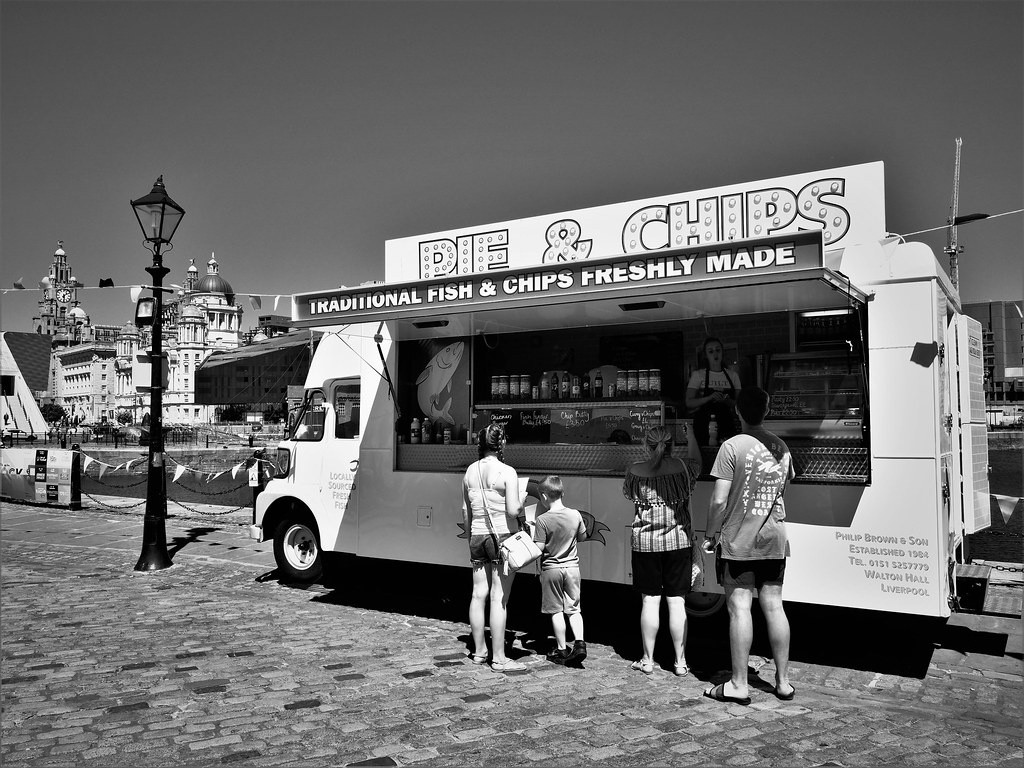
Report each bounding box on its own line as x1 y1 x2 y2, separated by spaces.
250 159 995 640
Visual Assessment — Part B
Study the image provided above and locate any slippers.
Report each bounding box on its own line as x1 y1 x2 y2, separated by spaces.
774 683 796 699
704 682 752 705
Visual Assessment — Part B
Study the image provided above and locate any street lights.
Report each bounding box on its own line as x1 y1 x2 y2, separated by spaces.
129 175 186 572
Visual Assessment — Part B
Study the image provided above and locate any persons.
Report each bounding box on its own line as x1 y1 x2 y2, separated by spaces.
462 423 527 671
533 475 587 665
686 337 741 446
702 386 796 705
623 421 702 677
4 413 9 425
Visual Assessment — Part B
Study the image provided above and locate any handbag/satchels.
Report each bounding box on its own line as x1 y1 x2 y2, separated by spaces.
499 530 543 577
689 536 707 591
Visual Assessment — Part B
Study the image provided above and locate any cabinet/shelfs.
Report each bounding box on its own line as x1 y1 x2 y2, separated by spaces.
755 348 869 419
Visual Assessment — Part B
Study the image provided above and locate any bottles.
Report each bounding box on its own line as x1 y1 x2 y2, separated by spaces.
410 417 452 446
491 368 661 403
708 415 723 445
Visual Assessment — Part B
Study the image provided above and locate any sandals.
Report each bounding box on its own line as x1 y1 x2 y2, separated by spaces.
472 649 492 665
492 657 527 673
630 659 654 674
674 664 690 676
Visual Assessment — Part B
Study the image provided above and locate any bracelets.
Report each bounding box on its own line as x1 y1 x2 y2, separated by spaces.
705 536 715 541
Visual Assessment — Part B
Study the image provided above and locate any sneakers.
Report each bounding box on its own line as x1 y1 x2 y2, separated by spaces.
546 649 567 663
565 641 587 666
